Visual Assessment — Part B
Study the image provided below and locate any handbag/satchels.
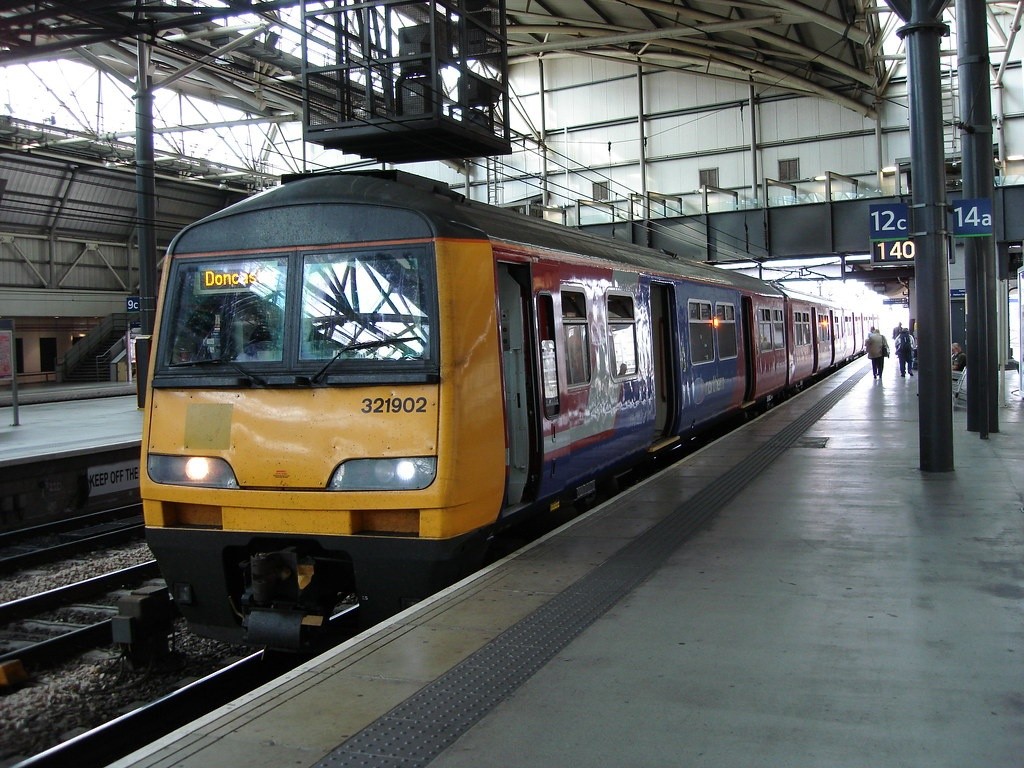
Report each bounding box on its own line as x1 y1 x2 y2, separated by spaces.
883 347 888 357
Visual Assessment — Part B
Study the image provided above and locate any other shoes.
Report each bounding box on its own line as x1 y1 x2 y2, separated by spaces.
908 371 913 376
874 375 877 378
879 374 882 377
901 373 905 377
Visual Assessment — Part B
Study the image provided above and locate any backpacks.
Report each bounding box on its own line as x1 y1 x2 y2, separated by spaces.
899 333 911 353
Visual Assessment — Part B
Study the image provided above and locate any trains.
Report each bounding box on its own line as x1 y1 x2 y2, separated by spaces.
137 166 891 653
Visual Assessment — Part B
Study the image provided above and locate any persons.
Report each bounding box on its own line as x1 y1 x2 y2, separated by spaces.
951 343 967 371
893 322 902 339
895 328 914 377
868 327 887 378
